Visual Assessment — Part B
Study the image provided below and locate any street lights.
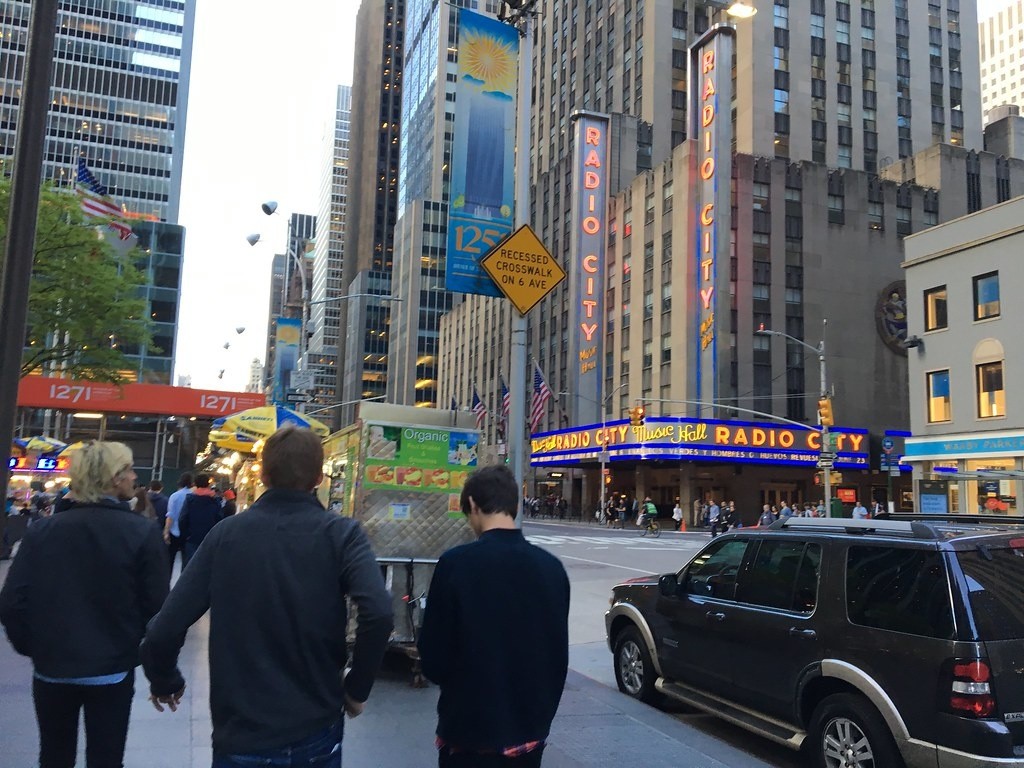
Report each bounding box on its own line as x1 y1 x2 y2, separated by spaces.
558 382 630 525
246 201 405 415
756 329 833 519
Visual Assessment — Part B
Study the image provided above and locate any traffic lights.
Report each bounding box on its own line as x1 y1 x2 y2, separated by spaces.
818 400 834 426
635 406 645 426
628 408 636 425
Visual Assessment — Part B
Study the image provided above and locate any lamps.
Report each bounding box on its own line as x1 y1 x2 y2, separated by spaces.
903 336 921 348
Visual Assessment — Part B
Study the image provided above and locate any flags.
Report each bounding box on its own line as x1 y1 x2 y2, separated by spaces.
77 158 133 241
530 368 550 434
500 381 510 419
470 388 485 428
451 398 456 409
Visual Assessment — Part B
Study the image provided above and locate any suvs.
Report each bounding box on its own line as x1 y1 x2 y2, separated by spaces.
604 513 1024 768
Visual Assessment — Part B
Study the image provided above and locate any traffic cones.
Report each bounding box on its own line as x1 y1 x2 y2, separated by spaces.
680 518 686 532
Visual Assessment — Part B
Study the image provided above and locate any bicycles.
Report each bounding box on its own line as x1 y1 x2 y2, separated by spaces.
638 513 661 538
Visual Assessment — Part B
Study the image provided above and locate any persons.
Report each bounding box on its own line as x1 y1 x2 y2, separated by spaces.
523 493 568 519
129 474 236 576
851 502 868 520
672 496 683 531
693 496 741 537
630 498 639 523
594 494 626 529
870 500 885 518
140 425 395 768
642 497 657 530
757 499 827 527
9 481 72 526
0 439 174 768
415 466 571 768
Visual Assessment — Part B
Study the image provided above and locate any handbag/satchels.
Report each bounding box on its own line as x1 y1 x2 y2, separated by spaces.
635 514 644 525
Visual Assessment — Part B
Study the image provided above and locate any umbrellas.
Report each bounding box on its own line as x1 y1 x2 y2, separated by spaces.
207 405 328 454
12 434 66 452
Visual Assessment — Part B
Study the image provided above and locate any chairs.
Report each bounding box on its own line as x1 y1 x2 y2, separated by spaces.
777 556 817 611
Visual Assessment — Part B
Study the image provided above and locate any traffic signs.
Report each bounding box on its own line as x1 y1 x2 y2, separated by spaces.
818 451 839 460
815 459 834 469
286 393 316 403
